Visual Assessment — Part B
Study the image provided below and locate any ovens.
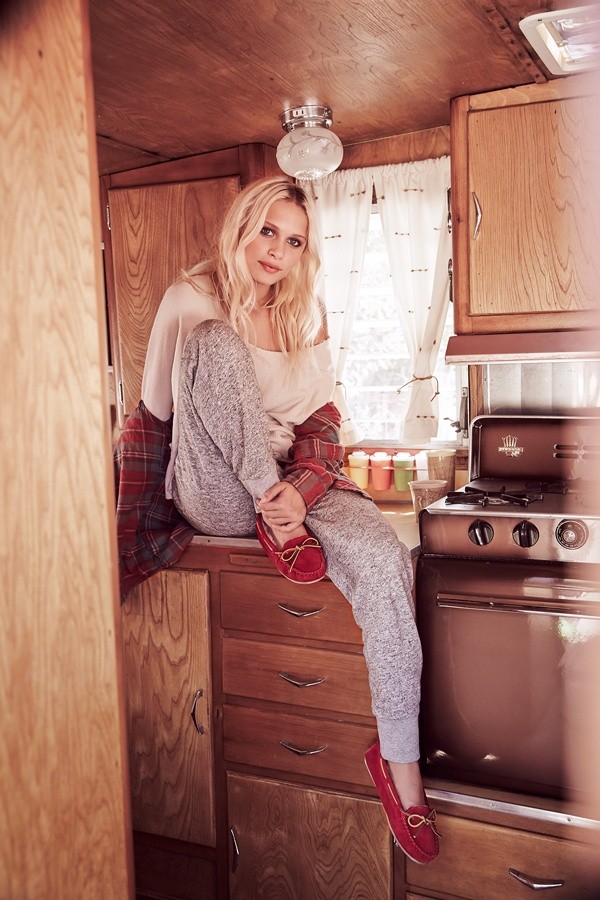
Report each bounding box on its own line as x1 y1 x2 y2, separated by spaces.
417 554 600 804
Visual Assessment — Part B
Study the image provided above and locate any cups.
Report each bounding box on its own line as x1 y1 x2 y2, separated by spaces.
408 479 448 523
426 449 457 493
392 453 415 492
369 451 392 491
416 450 428 480
348 450 369 490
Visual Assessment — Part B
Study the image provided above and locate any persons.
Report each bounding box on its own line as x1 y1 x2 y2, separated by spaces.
116 176 443 865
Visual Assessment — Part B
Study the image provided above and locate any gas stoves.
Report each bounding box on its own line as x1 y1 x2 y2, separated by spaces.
418 415 600 564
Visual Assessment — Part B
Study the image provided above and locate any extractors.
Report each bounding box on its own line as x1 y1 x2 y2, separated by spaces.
444 330 600 364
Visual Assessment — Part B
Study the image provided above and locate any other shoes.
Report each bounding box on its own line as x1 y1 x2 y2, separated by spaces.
254 512 326 585
362 740 443 865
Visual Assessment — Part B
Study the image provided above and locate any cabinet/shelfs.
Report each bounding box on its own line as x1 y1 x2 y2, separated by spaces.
119 551 600 900
445 64 600 334
101 144 284 435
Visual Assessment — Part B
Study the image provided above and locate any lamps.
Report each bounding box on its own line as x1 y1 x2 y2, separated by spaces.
273 100 348 188
